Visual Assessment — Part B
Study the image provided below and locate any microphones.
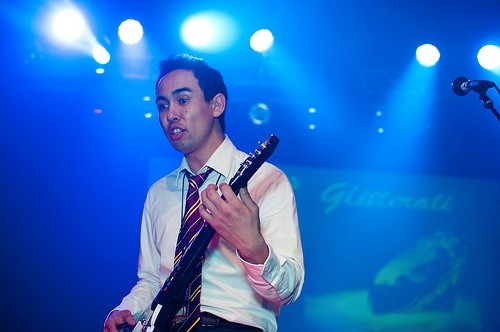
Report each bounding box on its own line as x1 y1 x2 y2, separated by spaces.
452 77 495 96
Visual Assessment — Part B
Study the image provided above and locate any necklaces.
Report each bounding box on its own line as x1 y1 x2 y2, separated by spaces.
181 172 222 220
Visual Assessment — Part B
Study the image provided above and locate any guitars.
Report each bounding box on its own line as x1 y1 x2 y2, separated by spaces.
117 133 280 332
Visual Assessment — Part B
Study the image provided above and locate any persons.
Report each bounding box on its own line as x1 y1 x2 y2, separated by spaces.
103 55 305 332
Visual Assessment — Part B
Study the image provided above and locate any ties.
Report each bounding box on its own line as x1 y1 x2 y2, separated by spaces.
173 168 214 332
